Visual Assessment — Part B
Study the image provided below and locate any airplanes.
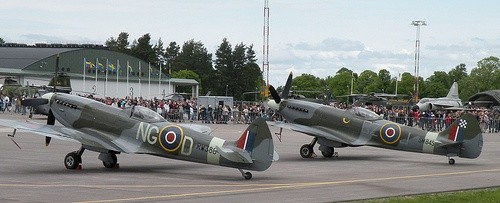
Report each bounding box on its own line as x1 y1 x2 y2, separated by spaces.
0 51 281 181
262 72 484 166
409 82 495 119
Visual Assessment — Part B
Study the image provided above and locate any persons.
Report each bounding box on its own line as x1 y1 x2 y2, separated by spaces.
333 102 500 133
85 95 289 124
0 91 41 115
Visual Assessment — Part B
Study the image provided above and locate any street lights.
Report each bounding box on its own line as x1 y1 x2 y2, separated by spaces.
409 20 427 102
347 85 350 105
292 85 297 94
226 85 228 97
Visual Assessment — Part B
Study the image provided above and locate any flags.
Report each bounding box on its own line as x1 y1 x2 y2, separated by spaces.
149 65 164 80
137 65 144 78
117 64 124 76
96 62 106 73
129 64 133 76
108 61 117 74
85 58 96 73
